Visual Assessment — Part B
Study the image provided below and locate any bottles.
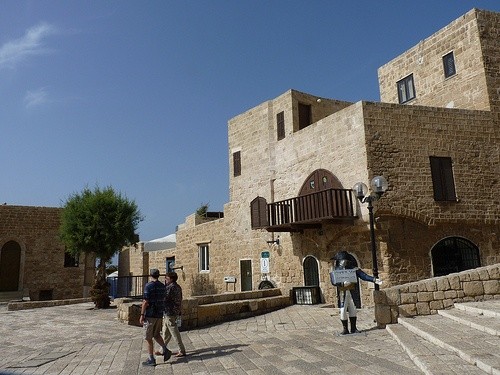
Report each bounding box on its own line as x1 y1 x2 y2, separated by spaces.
143 320 147 330
178 315 181 327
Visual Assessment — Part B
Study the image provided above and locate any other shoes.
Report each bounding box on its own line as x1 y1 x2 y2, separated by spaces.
142 358 156 366
155 351 164 355
176 353 186 356
164 348 172 362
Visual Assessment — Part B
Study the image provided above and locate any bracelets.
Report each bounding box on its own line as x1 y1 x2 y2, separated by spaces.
140 314 144 316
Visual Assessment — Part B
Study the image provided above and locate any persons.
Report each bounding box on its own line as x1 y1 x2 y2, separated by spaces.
154 273 186 357
329 250 382 335
138 269 172 366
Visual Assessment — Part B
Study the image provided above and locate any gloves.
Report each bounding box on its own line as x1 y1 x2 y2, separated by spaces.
343 281 351 287
375 278 383 285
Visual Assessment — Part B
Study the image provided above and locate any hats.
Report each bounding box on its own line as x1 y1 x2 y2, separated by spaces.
151 269 159 275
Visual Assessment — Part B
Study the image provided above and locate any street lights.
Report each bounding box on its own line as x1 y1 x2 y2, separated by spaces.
352 174 388 291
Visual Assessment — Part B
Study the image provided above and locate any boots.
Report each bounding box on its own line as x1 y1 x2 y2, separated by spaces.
349 316 360 333
340 319 349 334
